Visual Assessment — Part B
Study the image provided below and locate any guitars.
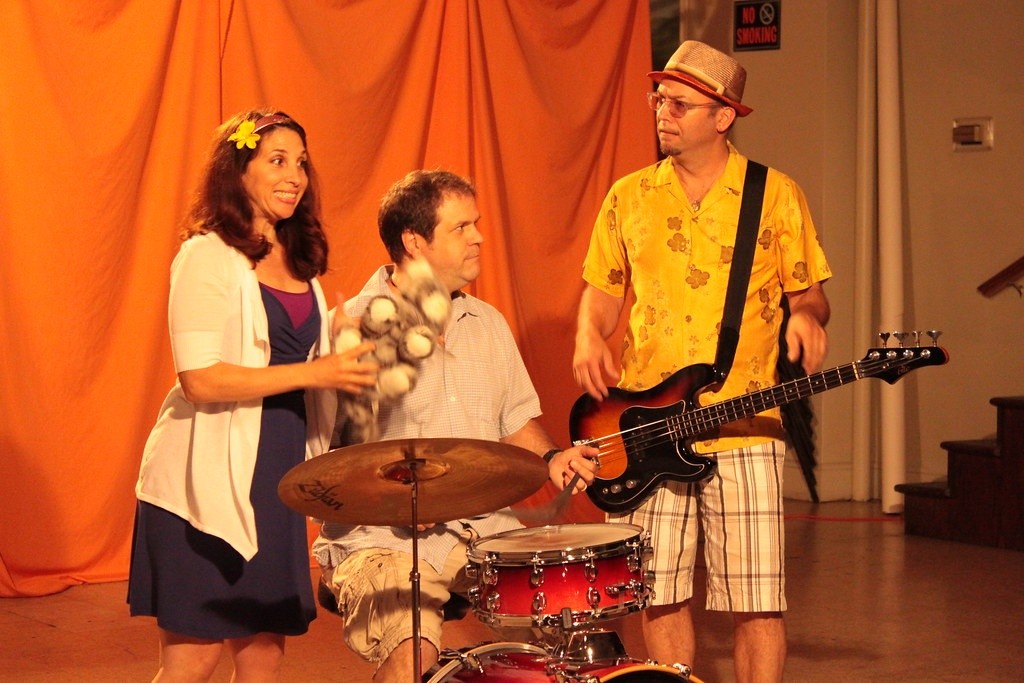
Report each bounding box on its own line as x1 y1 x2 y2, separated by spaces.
567 327 952 517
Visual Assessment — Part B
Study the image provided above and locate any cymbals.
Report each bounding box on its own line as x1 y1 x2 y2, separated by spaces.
276 435 551 526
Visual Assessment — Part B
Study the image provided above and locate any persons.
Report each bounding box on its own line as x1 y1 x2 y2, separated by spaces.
571 39 833 683
126 113 378 683
318 171 602 683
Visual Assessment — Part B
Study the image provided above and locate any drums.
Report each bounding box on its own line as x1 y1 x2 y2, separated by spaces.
421 640 704 683
465 522 653 630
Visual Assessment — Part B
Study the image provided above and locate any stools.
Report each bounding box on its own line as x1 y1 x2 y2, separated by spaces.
318 575 467 621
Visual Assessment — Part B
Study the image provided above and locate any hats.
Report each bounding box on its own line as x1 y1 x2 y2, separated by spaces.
646 39 753 119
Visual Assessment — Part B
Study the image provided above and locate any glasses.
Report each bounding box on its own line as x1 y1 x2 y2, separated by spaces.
646 92 724 118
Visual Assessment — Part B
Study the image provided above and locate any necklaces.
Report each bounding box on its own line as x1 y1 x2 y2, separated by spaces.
670 145 729 213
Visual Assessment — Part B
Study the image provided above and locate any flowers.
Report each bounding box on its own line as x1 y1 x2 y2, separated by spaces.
226 119 260 149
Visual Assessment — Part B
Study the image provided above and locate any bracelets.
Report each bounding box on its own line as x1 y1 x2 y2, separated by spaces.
541 448 563 482
